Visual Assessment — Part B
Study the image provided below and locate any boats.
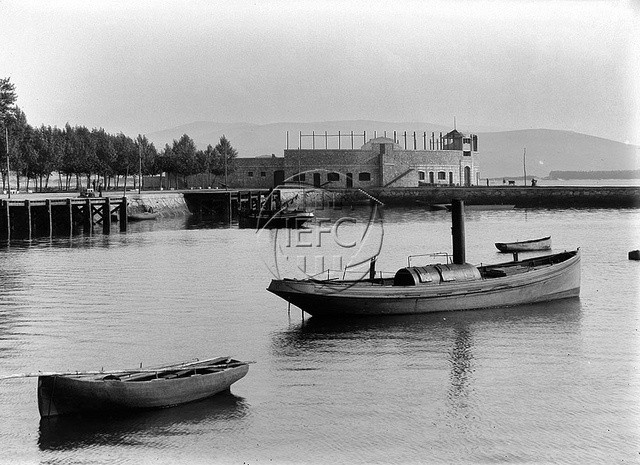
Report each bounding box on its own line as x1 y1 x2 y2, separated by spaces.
248 208 314 228
267 198 582 314
37 355 251 417
496 235 552 250
112 208 158 219
